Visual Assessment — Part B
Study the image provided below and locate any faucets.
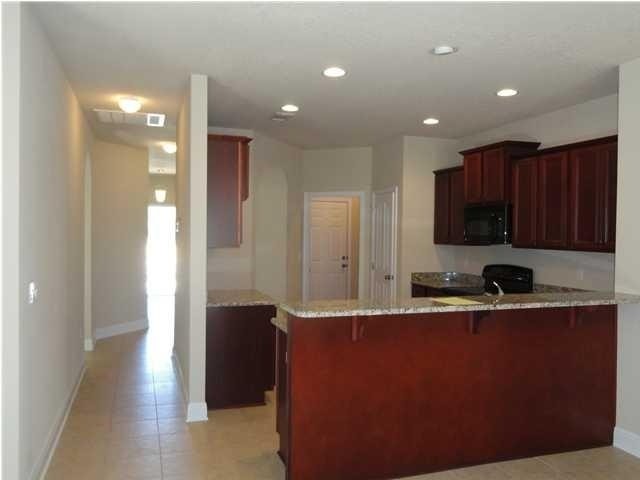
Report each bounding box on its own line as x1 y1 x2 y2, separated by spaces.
492 279 505 307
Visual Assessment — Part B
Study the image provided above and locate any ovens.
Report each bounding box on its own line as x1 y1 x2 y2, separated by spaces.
464 205 510 244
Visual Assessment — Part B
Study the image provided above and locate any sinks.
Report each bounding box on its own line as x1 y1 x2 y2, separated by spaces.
435 296 485 306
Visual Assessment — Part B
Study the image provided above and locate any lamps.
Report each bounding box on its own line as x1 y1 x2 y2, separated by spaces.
117 96 142 113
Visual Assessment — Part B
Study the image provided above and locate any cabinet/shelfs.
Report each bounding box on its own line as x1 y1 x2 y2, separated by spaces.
459 139 541 203
511 131 618 253
432 165 464 245
208 133 255 249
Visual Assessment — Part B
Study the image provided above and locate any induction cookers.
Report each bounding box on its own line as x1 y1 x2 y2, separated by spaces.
445 283 529 294
